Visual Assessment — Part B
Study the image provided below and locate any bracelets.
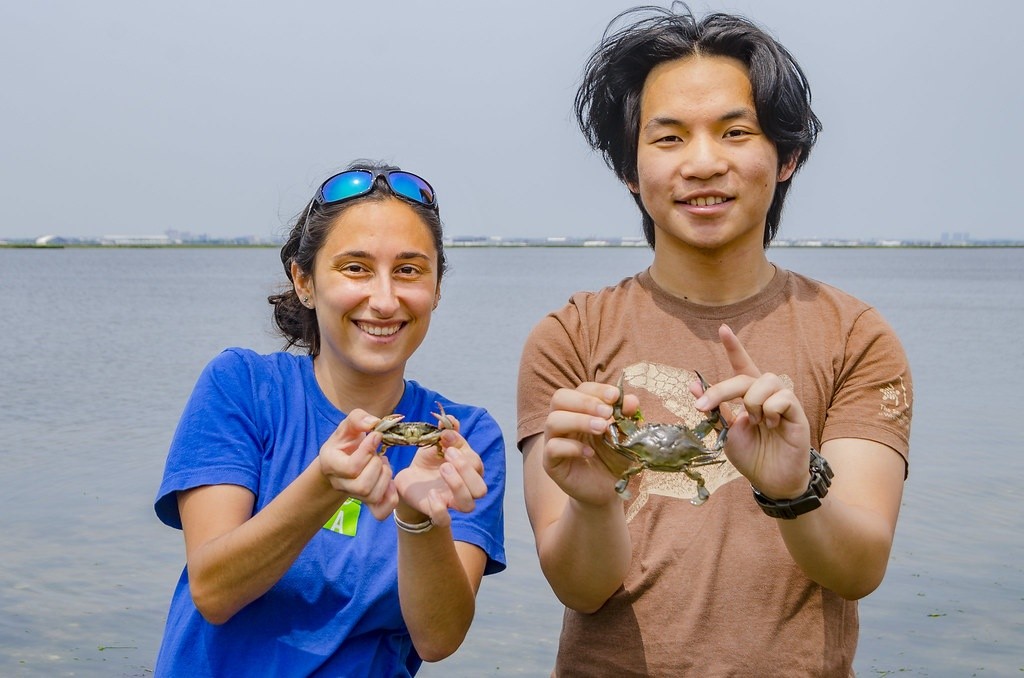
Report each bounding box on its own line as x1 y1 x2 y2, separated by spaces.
394 509 435 534
751 447 834 519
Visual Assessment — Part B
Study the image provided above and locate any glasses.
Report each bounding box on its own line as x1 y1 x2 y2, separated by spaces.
296 166 442 263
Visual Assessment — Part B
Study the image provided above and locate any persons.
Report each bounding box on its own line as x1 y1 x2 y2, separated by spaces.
154 156 507 678
517 0 913 677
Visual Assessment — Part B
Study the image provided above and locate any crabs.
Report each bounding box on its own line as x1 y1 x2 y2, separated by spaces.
369 400 455 459
602 371 729 507
